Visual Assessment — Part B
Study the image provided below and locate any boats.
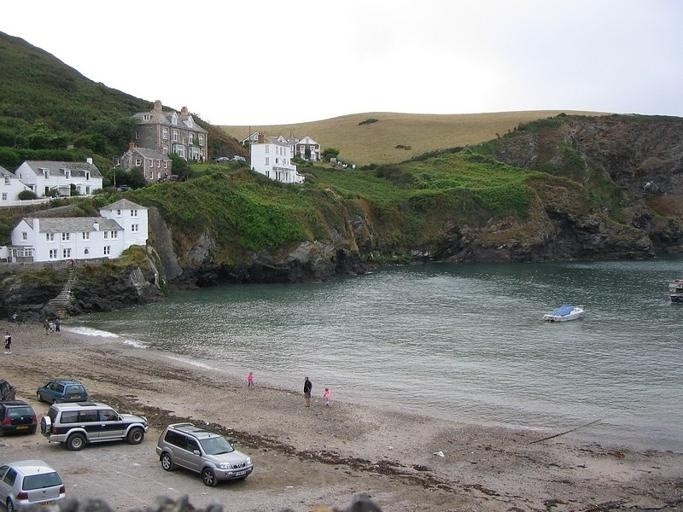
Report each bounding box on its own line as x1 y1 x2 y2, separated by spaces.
668 279 683 302
543 307 585 322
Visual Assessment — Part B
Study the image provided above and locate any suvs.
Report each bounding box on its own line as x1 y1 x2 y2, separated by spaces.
41 402 149 451
156 422 254 487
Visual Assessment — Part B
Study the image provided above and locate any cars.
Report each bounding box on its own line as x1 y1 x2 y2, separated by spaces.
0 379 37 436
37 380 88 404
1 460 66 512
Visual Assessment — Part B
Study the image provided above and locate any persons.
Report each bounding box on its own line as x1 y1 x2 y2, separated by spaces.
48 320 53 333
54 317 59 332
9 312 18 320
322 388 329 406
0 330 9 351
3 332 12 354
42 318 49 335
247 372 255 388
302 376 312 407
7 312 16 323
